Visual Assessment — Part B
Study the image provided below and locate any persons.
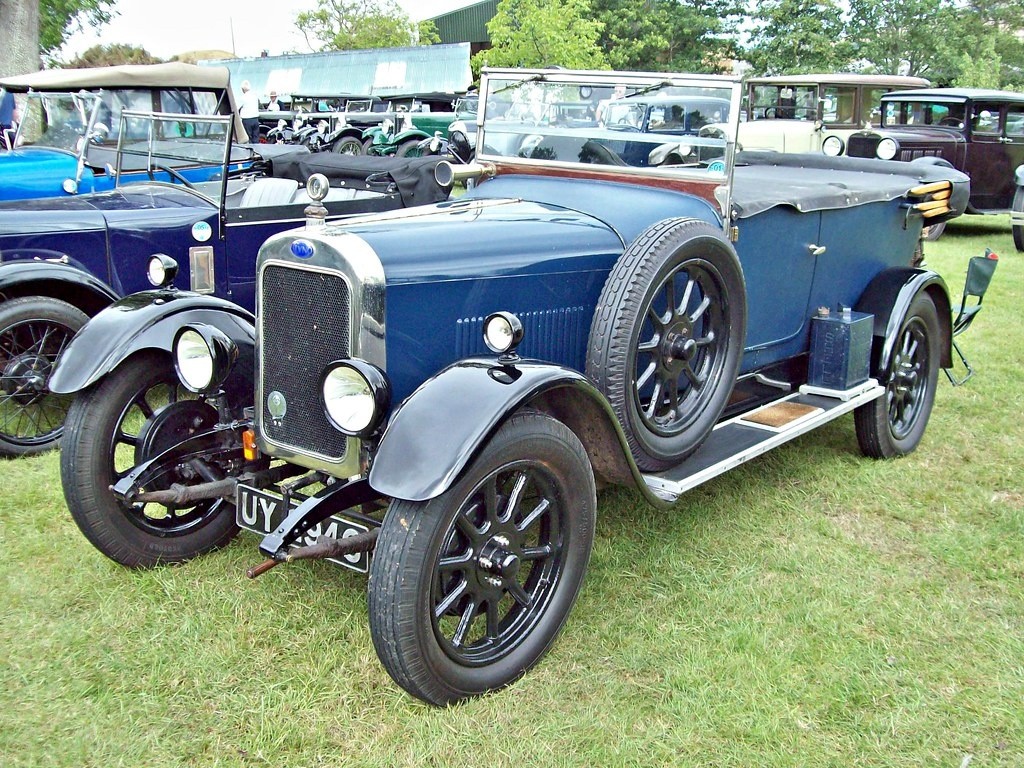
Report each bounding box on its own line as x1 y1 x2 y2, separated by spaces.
599 84 635 128
0 86 16 130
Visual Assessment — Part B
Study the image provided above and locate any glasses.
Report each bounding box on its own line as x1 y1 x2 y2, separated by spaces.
615 90 622 94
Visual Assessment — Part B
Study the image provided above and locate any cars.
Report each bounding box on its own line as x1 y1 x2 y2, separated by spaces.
0 61 311 200
0 112 456 457
988 116 1024 132
256 92 741 170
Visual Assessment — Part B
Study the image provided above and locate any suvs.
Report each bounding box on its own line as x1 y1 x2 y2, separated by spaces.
695 74 930 161
814 87 1024 253
45 64 971 709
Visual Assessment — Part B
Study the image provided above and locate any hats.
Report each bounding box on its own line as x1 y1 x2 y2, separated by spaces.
267 91 278 96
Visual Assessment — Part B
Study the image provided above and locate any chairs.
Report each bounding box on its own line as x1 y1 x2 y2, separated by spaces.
239 174 298 210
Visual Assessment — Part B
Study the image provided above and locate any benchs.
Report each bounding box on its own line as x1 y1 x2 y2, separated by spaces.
843 109 913 125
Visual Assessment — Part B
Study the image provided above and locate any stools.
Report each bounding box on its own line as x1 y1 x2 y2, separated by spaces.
941 247 999 387
